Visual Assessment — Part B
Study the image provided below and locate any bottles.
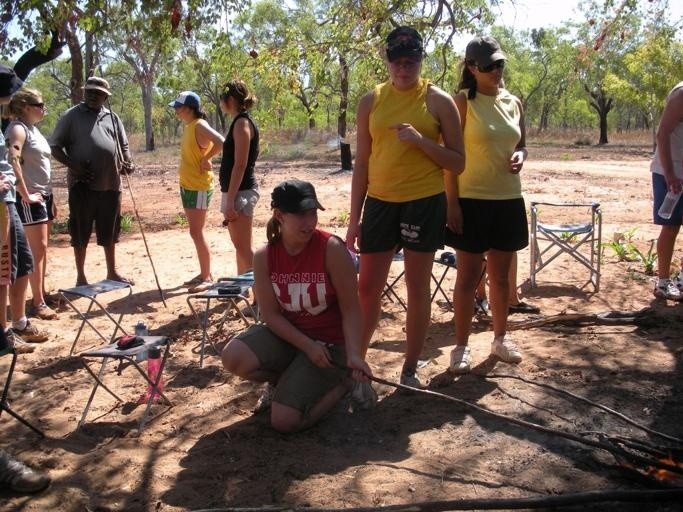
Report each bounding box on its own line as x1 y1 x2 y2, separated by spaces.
444 254 456 266
658 180 682 220
134 320 148 362
146 347 165 398
222 197 248 226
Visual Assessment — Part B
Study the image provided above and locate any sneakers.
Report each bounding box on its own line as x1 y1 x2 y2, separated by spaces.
653 280 683 301
474 298 488 313
119 277 135 287
491 339 522 363
509 301 539 313
0 328 36 353
188 282 213 293
254 386 275 414
353 379 377 411
0 448 51 493
400 373 420 394
11 320 50 342
29 298 57 320
184 273 213 286
450 347 470 374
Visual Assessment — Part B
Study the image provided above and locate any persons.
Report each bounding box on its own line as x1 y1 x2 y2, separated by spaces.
217 82 260 277
475 249 541 315
49 76 138 286
346 27 466 396
2 191 16 356
444 37 528 376
12 88 58 320
649 81 683 302
221 179 373 435
166 92 226 294
0 68 50 354
0 438 52 496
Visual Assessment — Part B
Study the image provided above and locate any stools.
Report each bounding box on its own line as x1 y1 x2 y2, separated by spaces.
186 273 259 369
431 254 483 310
59 279 135 355
364 249 407 310
1 348 46 440
76 335 172 437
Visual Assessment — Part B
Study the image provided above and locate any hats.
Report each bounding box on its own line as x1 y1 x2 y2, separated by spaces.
0 65 23 97
466 36 508 68
168 91 201 108
386 26 422 62
80 77 112 96
271 180 326 213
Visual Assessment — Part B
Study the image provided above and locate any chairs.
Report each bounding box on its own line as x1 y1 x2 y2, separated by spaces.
530 199 602 293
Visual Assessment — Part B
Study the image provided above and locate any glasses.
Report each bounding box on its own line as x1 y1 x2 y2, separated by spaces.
28 103 44 107
472 60 505 72
